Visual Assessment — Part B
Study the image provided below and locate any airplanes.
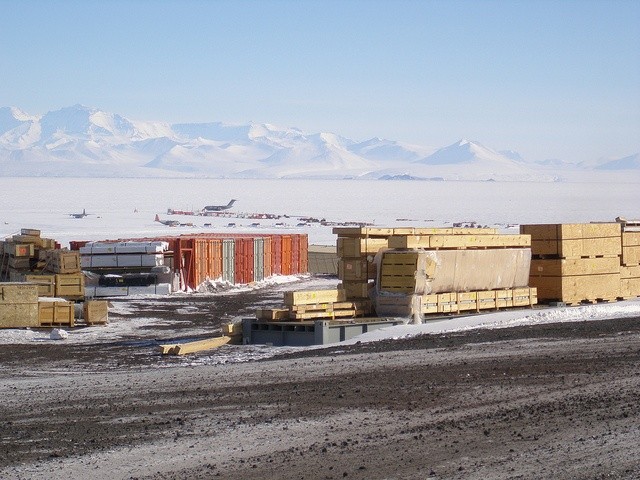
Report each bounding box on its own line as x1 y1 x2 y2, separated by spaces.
66 208 93 218
155 214 180 225
205 199 236 211
